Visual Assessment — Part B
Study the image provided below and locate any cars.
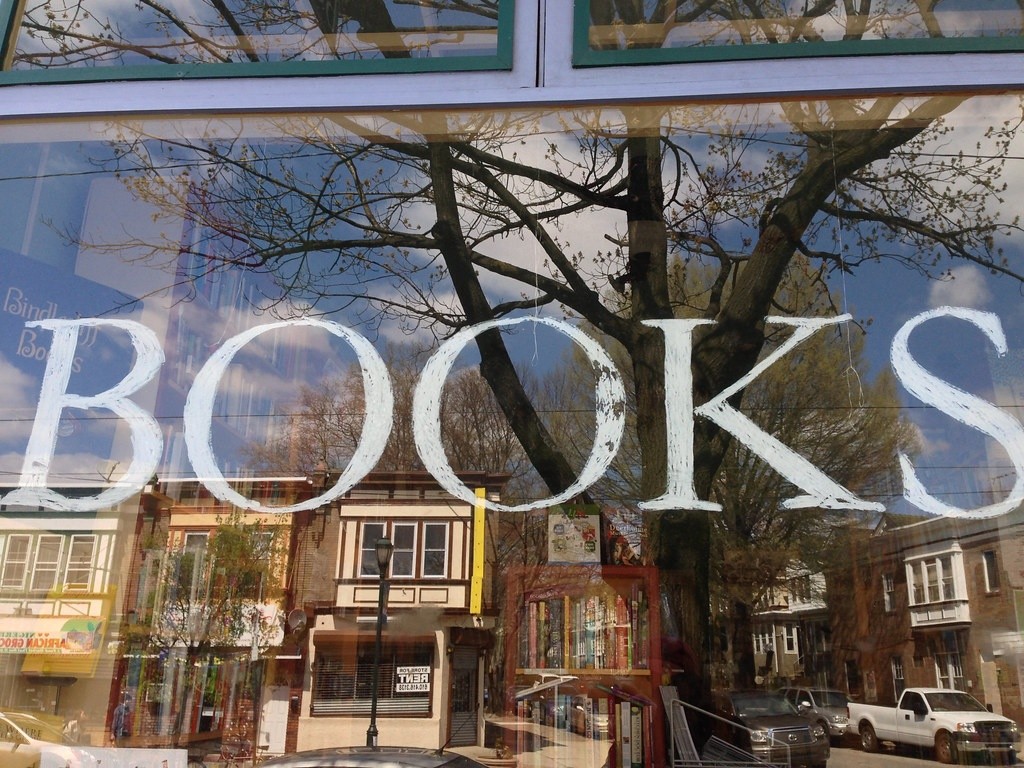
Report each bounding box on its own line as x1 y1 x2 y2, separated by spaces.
252 743 485 768
512 683 610 740
1 711 100 768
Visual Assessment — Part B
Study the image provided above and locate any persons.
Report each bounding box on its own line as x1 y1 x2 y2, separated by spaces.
62 709 86 747
109 694 137 748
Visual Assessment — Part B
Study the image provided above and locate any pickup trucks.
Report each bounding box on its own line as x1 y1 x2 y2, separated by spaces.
847 687 1023 767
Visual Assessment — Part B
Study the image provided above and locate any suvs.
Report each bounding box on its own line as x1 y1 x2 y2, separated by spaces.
713 687 833 768
775 682 854 744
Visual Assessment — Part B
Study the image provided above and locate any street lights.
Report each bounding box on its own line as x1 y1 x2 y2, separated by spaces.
364 537 393 747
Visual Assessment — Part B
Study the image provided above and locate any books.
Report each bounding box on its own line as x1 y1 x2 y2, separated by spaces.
123 197 316 739
513 499 649 766
